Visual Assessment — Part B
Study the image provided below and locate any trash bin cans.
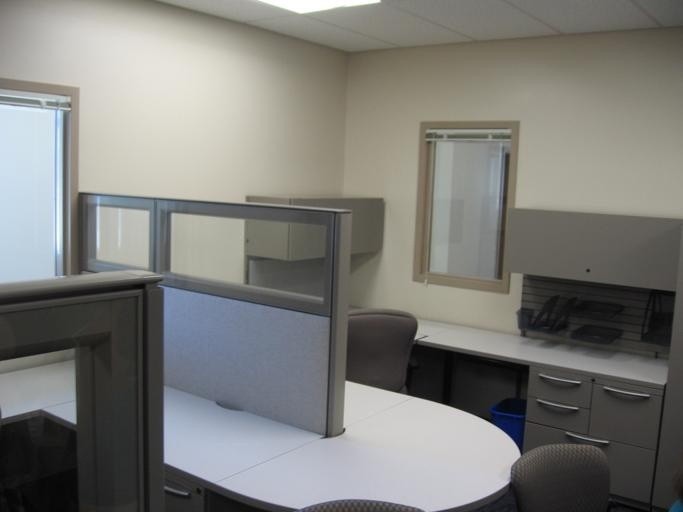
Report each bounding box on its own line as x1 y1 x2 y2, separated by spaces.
489 398 526 456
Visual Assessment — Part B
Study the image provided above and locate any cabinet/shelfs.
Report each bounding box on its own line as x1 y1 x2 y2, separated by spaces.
1 195 682 512
